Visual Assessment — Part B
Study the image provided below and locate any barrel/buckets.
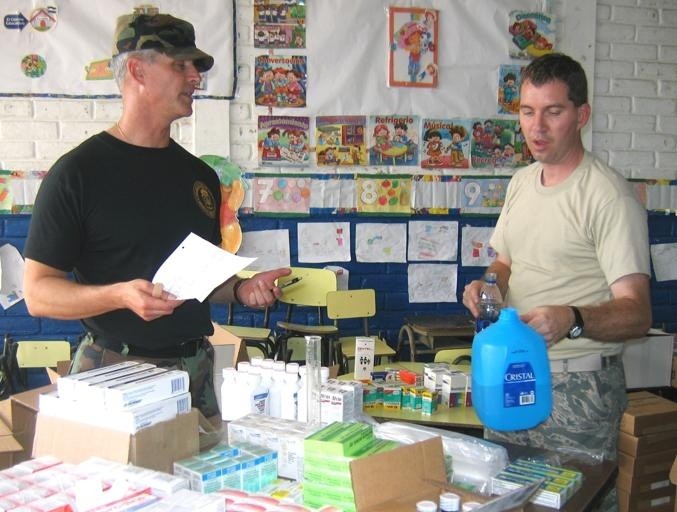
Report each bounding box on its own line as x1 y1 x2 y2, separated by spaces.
471 308 554 432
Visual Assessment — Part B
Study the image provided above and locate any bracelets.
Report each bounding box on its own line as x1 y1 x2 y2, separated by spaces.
233 277 246 309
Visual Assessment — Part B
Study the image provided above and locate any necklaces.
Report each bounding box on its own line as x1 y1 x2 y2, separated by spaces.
115 119 130 143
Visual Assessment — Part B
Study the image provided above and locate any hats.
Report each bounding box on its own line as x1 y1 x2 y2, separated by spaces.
112 13 214 72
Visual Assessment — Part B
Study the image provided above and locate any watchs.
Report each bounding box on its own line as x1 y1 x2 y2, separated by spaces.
569 306 583 340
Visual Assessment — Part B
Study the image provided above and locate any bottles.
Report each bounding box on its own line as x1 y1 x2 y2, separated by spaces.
415 491 483 512
304 334 322 423
473 272 505 338
221 356 329 422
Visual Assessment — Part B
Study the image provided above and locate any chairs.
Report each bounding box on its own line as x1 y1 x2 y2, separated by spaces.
14 340 71 390
219 266 473 379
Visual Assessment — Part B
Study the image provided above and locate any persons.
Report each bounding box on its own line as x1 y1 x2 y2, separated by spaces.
461 52 654 512
24 13 291 420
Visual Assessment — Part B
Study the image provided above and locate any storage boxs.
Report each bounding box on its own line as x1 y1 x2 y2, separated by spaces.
623 328 674 390
205 320 249 414
614 388 677 511
350 436 547 512
0 360 220 474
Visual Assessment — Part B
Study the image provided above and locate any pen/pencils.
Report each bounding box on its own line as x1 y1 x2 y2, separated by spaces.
242 277 302 308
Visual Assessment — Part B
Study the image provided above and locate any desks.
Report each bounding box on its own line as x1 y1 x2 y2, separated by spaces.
200 413 618 512
337 360 485 439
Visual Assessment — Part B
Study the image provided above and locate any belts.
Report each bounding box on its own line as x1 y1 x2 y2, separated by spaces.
94 335 204 357
550 355 616 373
